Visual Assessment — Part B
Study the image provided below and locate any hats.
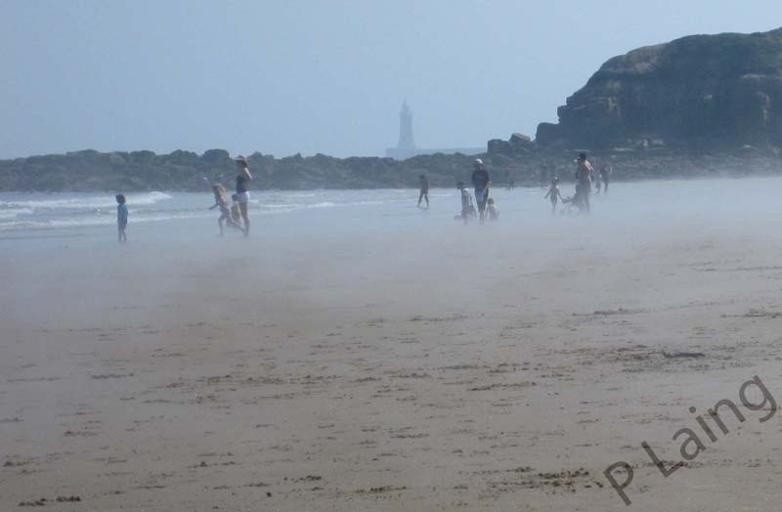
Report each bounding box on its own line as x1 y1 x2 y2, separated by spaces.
472 158 484 166
234 153 246 162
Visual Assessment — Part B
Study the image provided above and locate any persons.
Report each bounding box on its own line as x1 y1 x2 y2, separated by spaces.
116 194 128 242
417 175 429 208
209 184 245 236
457 151 613 223
234 155 253 236
229 194 243 225
